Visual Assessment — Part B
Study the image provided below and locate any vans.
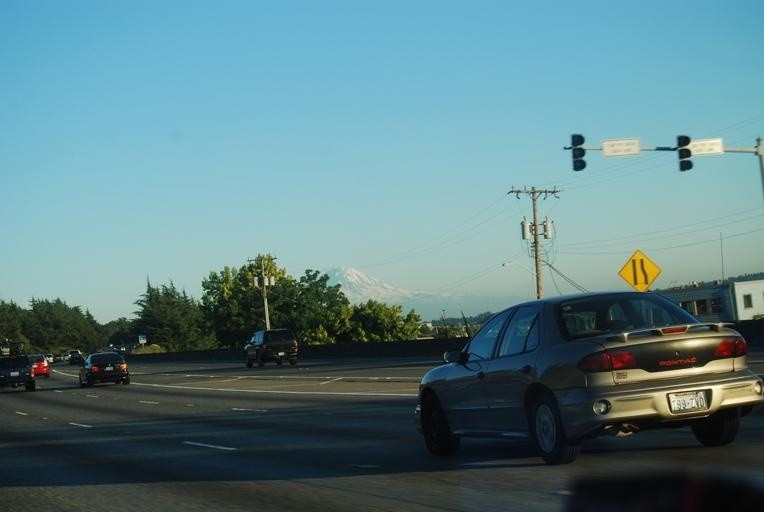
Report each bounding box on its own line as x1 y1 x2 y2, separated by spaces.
243 329 298 367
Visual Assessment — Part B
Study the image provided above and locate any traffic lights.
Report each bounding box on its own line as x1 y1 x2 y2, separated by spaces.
678 136 693 171
572 134 587 171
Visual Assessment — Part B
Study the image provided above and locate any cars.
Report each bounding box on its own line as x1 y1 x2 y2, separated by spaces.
0 344 129 391
415 292 764 465
